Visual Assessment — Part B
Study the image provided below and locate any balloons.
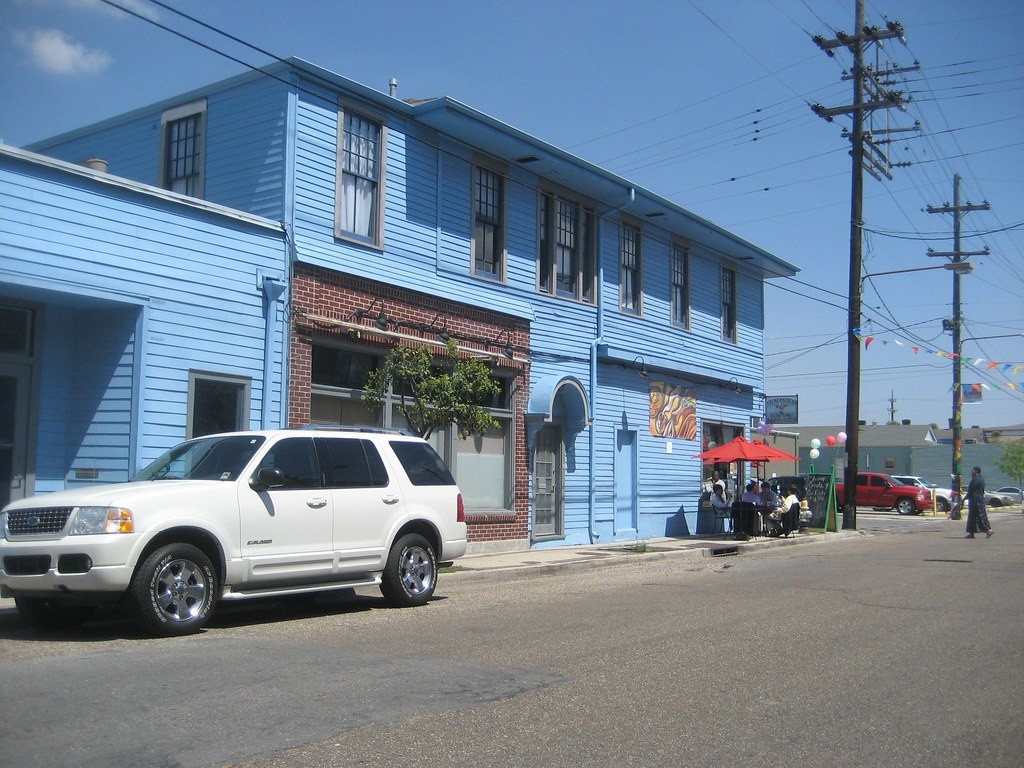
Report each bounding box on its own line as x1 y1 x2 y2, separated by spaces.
708 442 717 451
837 432 847 442
703 439 709 451
826 436 835 446
810 438 821 449
757 421 765 435
810 449 819 459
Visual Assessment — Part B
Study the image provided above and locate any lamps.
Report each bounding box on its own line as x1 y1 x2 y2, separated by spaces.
624 356 647 377
483 329 514 361
720 377 741 394
420 311 450 343
360 295 390 332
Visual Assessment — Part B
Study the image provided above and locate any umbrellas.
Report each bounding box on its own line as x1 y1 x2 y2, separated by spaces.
750 440 801 489
691 435 780 501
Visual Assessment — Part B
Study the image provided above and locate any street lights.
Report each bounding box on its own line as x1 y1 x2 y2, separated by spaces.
844 260 973 534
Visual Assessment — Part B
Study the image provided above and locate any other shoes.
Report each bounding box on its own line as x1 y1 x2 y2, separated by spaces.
985 529 994 538
964 533 975 538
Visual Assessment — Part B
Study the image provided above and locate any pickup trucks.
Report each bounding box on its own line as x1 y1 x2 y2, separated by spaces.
835 471 933 516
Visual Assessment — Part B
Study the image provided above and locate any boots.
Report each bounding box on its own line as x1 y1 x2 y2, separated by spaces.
775 524 785 537
767 528 777 537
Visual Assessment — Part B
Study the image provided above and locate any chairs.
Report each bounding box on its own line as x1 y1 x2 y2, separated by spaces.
723 501 754 540
773 503 800 538
712 504 733 534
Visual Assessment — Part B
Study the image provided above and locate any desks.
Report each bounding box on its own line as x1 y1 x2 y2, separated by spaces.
734 507 768 537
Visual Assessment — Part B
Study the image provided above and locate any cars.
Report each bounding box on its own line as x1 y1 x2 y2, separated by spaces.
962 485 1024 508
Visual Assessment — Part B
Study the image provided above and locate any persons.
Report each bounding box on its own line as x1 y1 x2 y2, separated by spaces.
960 467 994 538
706 469 726 493
765 488 799 537
710 481 777 537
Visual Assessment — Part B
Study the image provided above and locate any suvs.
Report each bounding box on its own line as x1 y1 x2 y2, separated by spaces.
1 426 468 637
889 475 952 512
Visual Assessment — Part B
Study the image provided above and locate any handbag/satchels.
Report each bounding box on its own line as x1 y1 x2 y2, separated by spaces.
949 501 963 520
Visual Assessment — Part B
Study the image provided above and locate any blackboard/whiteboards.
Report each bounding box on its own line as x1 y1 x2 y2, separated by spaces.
799 473 837 532
